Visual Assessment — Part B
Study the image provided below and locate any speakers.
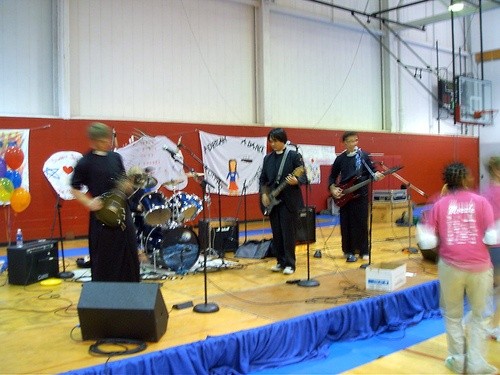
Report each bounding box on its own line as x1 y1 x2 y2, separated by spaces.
77 281 169 343
7 240 59 286
297 206 316 246
211 226 239 253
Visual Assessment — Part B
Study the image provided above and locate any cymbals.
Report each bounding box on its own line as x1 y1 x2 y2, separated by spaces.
162 180 183 185
182 172 205 177
129 175 158 189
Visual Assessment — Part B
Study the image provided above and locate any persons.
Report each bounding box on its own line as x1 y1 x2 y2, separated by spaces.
478 155 500 342
259 128 309 274
69 122 167 283
328 131 386 262
416 161 500 375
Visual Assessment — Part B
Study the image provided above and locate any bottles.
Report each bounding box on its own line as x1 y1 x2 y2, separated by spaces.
17 229 22 248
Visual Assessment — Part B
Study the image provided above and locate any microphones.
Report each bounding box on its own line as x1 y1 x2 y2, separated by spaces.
354 146 362 157
176 136 182 148
163 146 176 156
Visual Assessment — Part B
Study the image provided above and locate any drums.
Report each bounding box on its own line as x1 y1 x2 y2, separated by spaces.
146 222 201 273
168 191 197 223
139 191 171 226
190 194 203 222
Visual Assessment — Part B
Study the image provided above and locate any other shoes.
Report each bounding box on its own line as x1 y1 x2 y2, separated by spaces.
445 355 465 374
270 263 282 272
465 360 499 375
344 253 355 258
283 266 295 275
361 254 369 261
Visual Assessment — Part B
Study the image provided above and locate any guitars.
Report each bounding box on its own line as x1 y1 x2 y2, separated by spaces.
258 165 304 216
332 165 404 207
93 166 143 228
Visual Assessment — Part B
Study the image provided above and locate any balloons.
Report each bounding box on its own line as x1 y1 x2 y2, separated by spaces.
10 187 31 216
0 177 14 205
0 146 24 192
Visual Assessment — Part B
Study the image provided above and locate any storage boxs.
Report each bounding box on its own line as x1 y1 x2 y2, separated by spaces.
372 189 414 225
366 262 407 291
